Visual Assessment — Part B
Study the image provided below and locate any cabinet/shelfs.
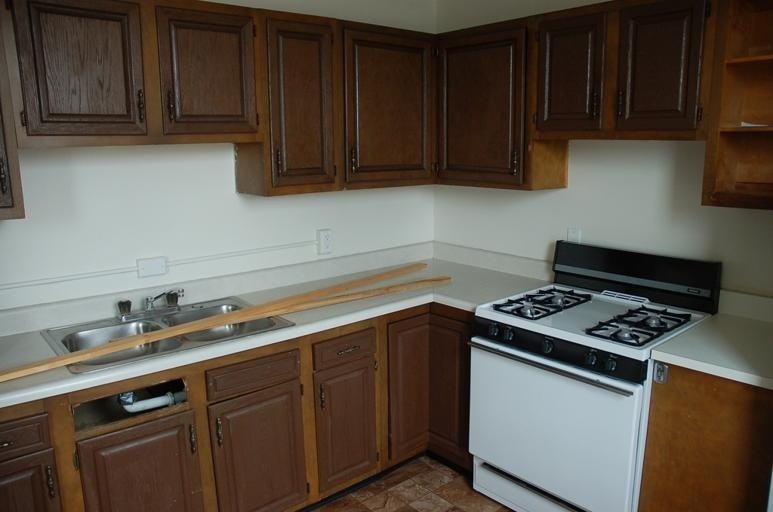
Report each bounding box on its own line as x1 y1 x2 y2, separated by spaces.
233 10 434 197
433 4 569 191
305 317 381 506
58 337 304 512
0 385 63 512
379 300 475 474
0 51 25 222
697 0 772 210
636 363 772 511
527 0 702 138
0 0 268 146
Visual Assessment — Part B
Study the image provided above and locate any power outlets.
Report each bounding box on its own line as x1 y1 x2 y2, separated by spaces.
316 228 332 256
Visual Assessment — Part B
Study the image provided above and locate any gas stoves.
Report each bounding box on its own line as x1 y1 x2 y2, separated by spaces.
472 280 712 383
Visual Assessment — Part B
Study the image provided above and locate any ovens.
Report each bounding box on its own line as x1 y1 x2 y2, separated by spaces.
469 337 652 510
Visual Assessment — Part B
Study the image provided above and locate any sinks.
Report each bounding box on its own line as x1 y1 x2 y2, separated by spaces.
158 295 296 348
40 316 188 375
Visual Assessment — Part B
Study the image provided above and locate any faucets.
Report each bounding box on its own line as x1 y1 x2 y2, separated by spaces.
145 287 184 311
